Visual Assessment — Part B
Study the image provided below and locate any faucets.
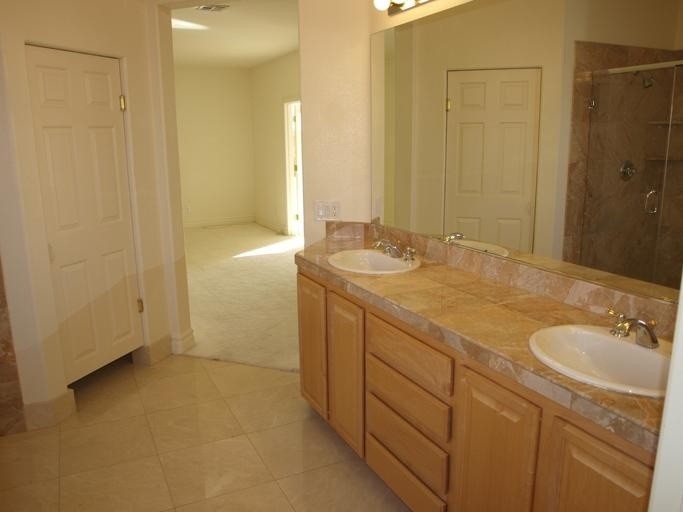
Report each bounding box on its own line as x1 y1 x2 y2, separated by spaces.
609 320 661 349
445 232 464 242
374 237 404 258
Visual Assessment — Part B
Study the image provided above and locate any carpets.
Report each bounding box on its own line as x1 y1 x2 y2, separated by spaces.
182 222 303 372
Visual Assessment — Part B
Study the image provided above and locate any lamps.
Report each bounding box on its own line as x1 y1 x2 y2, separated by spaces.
372 0 433 17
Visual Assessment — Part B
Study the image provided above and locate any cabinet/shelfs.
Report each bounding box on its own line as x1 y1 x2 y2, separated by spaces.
450 348 540 512
365 302 452 512
532 390 657 512
293 265 365 459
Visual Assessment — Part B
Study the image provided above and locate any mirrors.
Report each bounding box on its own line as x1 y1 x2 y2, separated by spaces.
367 1 683 305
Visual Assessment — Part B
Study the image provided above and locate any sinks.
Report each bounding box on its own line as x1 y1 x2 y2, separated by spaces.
527 322 670 400
456 239 509 258
327 247 422 276
